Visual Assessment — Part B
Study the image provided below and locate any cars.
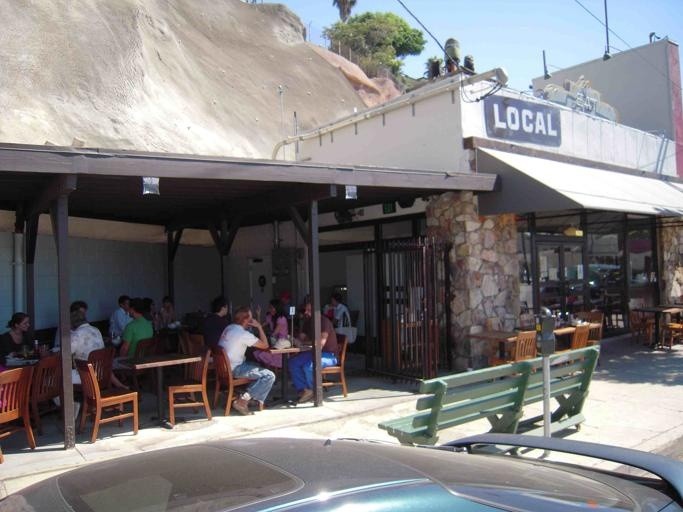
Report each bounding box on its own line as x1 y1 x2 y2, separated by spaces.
514 263 650 314
0 432 683 510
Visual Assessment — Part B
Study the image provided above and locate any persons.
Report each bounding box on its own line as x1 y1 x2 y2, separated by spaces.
0 312 34 359
51 300 90 408
109 291 353 417
63 310 105 421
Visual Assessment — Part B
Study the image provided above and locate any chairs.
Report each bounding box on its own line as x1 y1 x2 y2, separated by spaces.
608 299 683 352
319 334 349 397
572 323 589 351
490 330 539 374
74 347 115 393
74 359 139 446
0 366 35 462
1 325 211 364
30 352 64 436
212 345 264 416
578 310 605 345
165 349 212 424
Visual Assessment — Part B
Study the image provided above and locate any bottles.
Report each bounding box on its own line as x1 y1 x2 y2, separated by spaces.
32 339 41 358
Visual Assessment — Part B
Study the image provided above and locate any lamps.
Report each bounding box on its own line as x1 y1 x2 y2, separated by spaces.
602 44 623 61
344 185 357 200
559 207 583 238
542 62 566 81
142 176 161 201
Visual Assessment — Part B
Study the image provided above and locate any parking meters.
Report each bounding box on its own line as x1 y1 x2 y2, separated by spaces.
533 306 558 457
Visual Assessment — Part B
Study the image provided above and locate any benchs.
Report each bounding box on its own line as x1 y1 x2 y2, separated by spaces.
377 346 600 451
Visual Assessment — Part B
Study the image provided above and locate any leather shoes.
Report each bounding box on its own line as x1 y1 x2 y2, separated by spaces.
298 388 315 403
248 399 263 410
233 398 254 416
